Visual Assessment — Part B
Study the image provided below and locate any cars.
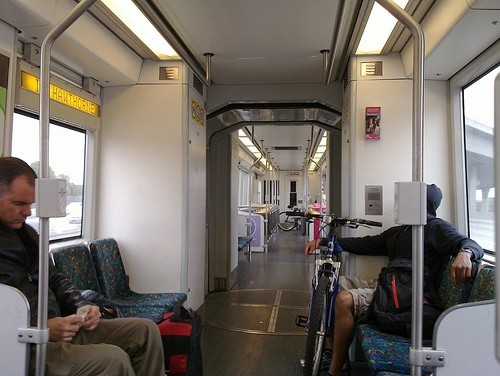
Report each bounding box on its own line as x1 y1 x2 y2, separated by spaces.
65 202 81 223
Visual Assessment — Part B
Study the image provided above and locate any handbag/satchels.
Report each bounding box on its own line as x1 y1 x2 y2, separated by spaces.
154 305 203 376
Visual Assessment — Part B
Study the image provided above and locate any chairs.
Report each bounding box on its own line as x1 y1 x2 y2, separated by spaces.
357 255 497 376
49 238 186 325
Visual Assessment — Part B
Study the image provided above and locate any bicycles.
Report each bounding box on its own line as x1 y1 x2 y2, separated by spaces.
277 205 383 376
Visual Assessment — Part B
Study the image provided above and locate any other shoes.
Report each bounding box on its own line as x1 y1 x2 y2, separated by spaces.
321 353 331 365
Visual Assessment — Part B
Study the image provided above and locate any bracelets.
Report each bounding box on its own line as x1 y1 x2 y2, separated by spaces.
459 249 474 257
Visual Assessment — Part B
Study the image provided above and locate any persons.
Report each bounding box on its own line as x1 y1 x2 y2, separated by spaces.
306 184 484 376
0 156 164 376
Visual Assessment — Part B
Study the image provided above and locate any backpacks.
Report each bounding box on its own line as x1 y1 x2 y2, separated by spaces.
367 256 435 336
